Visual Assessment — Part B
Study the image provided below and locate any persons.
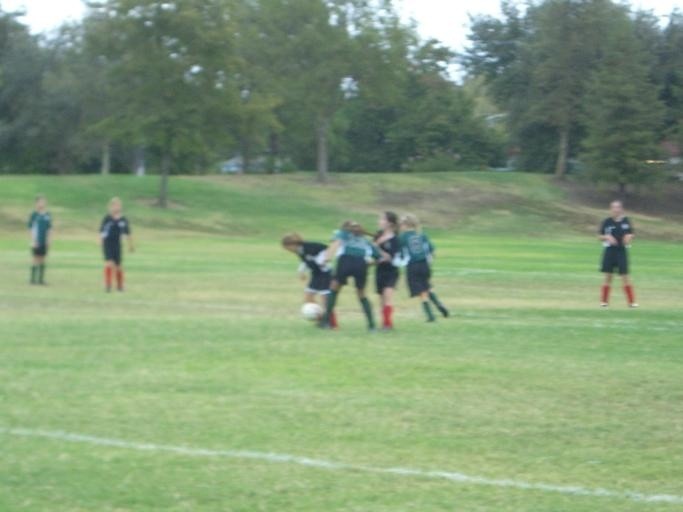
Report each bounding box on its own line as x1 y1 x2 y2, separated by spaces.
96 197 136 293
26 194 52 287
281 209 449 330
594 200 639 308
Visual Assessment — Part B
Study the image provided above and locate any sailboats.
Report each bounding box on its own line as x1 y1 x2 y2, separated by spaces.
303 302 323 321
